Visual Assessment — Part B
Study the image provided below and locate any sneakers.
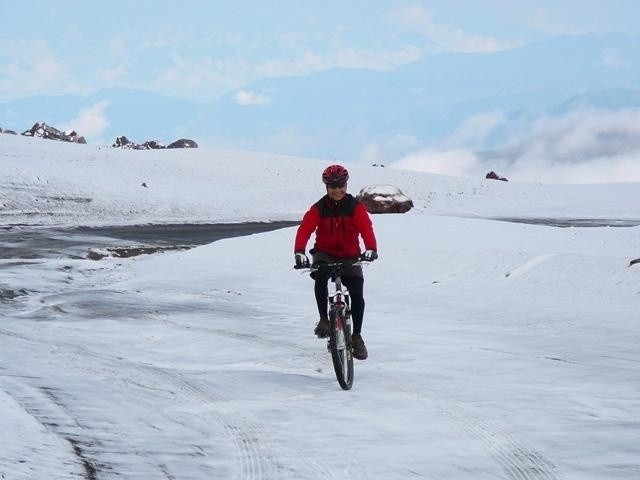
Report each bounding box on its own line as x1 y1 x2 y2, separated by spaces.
315 319 329 335
351 334 368 359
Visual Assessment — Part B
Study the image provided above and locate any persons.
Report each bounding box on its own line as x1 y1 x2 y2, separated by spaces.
294 165 378 360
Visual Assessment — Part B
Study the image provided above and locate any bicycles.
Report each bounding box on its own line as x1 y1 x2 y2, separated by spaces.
294 250 378 390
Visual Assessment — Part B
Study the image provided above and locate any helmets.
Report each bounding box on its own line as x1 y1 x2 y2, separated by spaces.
323 165 349 184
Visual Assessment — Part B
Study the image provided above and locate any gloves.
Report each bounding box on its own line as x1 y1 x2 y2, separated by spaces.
295 252 310 268
361 250 376 261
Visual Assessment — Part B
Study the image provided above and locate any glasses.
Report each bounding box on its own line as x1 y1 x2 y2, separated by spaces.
330 184 346 188
